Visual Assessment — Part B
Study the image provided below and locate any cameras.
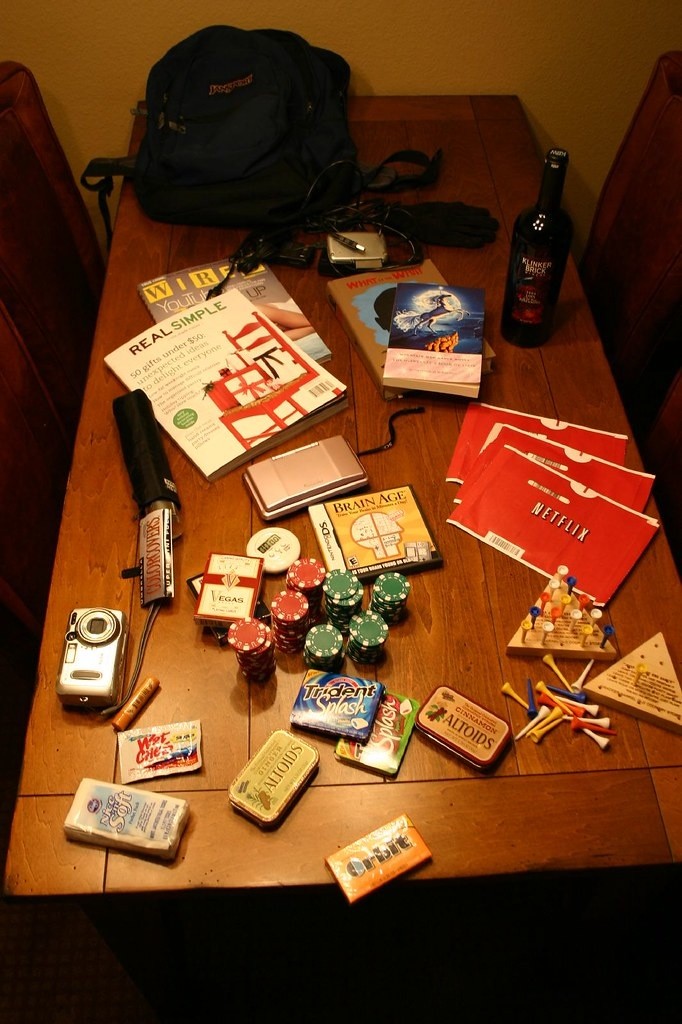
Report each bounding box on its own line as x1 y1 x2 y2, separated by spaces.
54 607 129 709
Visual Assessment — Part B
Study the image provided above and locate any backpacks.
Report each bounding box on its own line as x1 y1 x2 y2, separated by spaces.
79 24 444 252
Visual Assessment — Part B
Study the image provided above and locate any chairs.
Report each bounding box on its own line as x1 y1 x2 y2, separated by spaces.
0 59 101 632
580 47 680 556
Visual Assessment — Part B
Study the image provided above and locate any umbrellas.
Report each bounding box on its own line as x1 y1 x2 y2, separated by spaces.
111 388 183 543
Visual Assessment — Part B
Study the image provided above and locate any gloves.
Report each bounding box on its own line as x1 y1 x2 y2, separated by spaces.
375 202 499 249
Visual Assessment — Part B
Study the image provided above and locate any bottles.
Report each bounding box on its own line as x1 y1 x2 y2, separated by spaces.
227 558 411 684
498 147 574 349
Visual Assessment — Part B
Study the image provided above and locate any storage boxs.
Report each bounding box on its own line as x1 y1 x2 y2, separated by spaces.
192 551 263 625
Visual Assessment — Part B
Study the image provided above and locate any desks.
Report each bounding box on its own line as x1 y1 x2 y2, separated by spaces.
3 93 682 896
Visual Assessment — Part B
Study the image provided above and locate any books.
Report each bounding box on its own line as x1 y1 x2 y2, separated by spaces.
136 256 333 366
105 289 349 481
326 259 497 399
382 283 486 399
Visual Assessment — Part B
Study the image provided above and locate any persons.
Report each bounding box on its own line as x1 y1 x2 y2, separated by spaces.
207 289 315 341
